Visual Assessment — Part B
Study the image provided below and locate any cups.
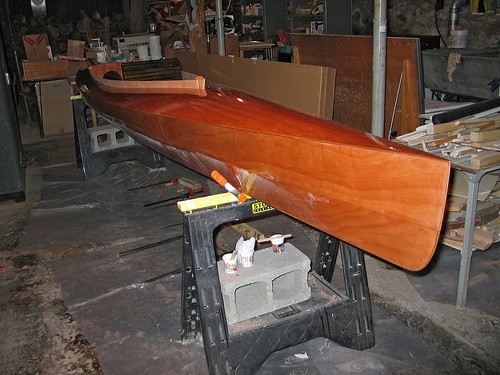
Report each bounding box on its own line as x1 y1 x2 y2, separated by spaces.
149 36 162 61
137 45 149 61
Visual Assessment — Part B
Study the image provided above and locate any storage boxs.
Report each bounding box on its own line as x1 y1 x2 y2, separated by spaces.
20 32 90 137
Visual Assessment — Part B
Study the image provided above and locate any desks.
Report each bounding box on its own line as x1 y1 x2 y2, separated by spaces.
390 111 500 310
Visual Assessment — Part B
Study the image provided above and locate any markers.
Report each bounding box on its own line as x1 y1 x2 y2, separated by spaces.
211 170 248 204
